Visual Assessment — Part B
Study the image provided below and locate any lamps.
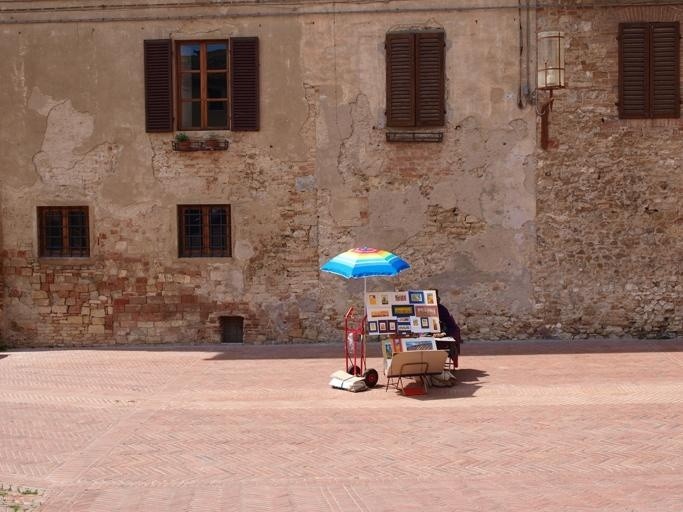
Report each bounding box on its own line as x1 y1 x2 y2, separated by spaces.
535 30 566 152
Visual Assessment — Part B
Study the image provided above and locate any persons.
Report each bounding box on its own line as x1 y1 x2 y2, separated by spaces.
428 288 455 349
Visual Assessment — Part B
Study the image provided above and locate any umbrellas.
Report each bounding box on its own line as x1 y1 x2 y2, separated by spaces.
320 245 411 341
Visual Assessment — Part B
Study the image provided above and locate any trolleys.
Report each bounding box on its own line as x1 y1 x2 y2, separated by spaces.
343 304 378 390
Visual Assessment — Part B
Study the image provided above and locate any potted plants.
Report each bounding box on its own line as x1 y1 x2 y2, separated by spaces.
176 134 191 149
